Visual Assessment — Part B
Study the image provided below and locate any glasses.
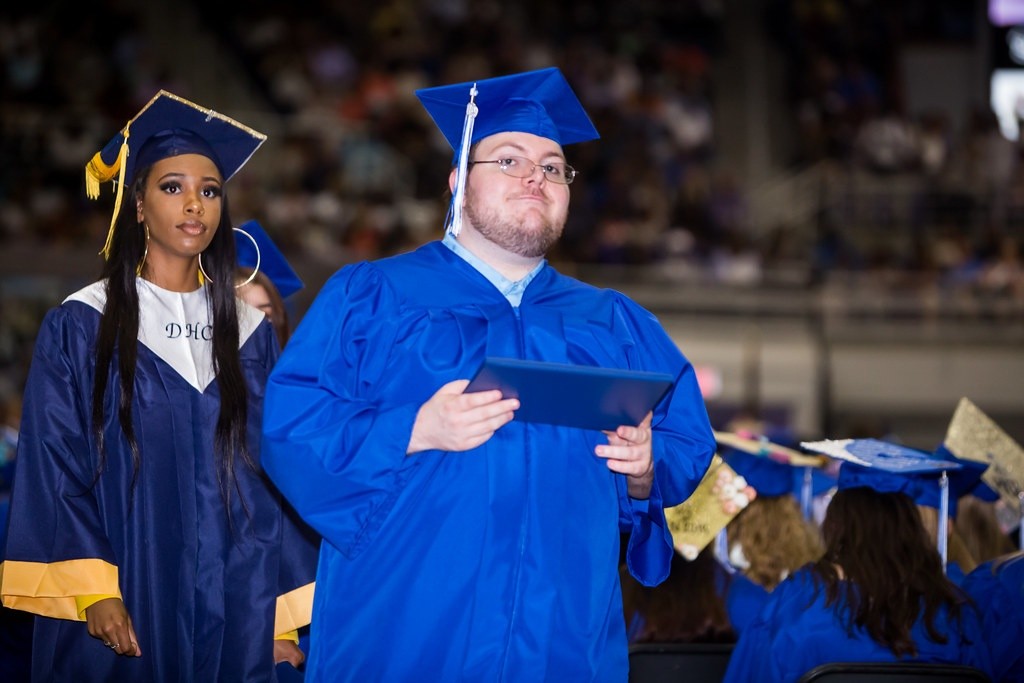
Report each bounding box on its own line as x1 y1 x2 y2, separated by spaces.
468 153 582 186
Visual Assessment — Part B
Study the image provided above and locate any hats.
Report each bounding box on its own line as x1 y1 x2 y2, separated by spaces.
83 87 268 263
711 427 837 577
232 217 305 302
413 64 602 238
796 435 1003 579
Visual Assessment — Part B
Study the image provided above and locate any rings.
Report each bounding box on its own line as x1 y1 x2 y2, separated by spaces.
104 640 111 646
111 643 120 650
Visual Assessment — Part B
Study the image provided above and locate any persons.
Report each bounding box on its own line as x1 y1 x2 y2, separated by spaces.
257 65 720 682
704 408 1024 677
224 266 289 356
1 0 1024 287
0 90 319 683
